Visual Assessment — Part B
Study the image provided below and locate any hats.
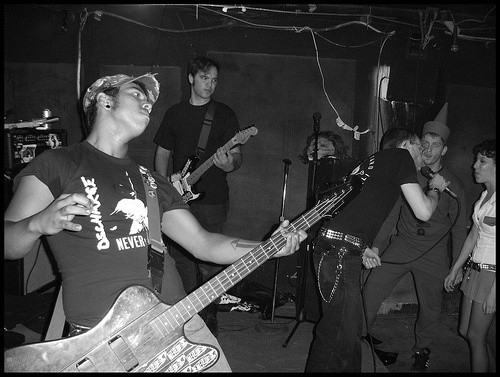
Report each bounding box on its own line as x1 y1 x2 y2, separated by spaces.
422 103 450 145
83 72 160 113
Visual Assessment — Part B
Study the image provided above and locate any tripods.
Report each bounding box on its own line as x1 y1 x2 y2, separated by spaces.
270 125 319 347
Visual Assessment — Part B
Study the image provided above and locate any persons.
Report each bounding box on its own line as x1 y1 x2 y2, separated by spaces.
305 128 449 371
444 139 497 373
361 132 467 370
4 74 308 373
153 56 243 337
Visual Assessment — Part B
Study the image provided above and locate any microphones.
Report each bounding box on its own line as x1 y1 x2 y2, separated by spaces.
420 166 458 199
312 112 322 129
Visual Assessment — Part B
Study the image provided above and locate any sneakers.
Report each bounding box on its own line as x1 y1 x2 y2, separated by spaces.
411 348 431 369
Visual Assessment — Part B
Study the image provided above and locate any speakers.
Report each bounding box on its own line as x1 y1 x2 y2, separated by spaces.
3 172 56 297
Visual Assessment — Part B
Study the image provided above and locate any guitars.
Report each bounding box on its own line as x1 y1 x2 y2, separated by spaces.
166 124 258 204
4 175 355 373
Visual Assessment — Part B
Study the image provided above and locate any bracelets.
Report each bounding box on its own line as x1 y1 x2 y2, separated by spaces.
427 184 439 192
